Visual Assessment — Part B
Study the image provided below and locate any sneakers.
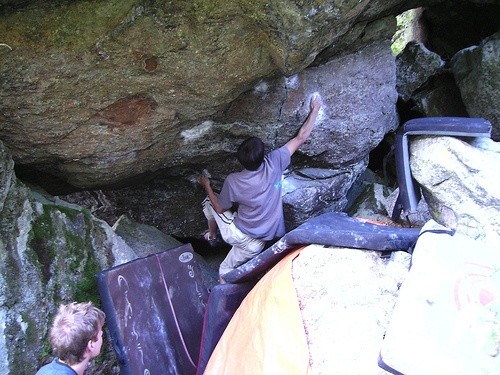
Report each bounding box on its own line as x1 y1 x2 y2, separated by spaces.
204 230 217 246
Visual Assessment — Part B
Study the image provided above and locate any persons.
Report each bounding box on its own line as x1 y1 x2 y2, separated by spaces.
197 95 322 284
35 301 106 375
